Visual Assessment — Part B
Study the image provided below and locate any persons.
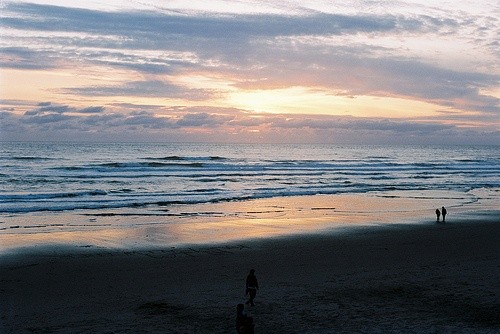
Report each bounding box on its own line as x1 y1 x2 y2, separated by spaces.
441 206 447 222
436 209 441 223
236 305 255 334
245 268 259 307
